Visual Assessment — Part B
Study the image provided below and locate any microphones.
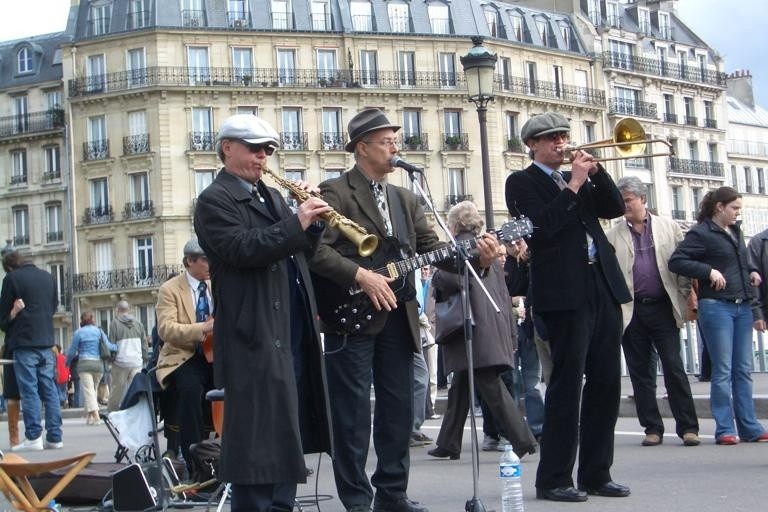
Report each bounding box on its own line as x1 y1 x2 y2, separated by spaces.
389 153 427 176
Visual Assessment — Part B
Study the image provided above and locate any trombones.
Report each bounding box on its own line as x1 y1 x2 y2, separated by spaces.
555 117 675 164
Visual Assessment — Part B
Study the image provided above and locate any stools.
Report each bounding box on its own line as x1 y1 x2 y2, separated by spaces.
0 453 95 512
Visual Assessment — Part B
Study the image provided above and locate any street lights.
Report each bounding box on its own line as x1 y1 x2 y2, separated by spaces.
461 30 501 234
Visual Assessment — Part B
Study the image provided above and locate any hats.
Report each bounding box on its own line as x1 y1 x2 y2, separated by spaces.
183 239 207 258
217 114 280 147
344 108 401 152
521 111 570 147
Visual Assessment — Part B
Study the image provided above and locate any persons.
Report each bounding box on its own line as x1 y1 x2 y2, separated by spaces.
410 201 546 460
304 107 503 512
607 176 768 445
0 239 219 470
192 112 336 511
504 110 635 502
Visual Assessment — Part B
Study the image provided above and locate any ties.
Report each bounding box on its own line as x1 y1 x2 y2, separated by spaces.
371 180 393 237
552 171 568 190
252 185 265 203
195 282 209 351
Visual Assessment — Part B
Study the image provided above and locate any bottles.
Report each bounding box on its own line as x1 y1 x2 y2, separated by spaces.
499 444 523 511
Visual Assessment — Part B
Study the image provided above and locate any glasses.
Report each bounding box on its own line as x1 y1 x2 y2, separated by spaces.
240 140 274 155
547 132 570 141
365 140 398 146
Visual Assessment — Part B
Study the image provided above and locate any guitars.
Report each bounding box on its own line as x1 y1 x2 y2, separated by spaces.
315 214 533 339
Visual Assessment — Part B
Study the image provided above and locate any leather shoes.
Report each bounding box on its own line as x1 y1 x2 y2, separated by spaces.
536 486 587 502
373 499 429 512
428 447 460 459
580 481 630 497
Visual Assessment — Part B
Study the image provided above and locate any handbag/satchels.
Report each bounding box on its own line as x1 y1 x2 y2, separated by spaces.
188 431 223 493
99 338 112 360
435 289 476 344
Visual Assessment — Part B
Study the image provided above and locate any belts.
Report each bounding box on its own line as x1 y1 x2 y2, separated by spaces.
722 298 751 304
636 296 670 305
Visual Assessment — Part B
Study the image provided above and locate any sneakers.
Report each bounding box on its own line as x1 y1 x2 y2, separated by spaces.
12 436 63 451
683 432 700 445
642 434 662 446
482 433 537 459
741 431 768 442
719 435 737 445
